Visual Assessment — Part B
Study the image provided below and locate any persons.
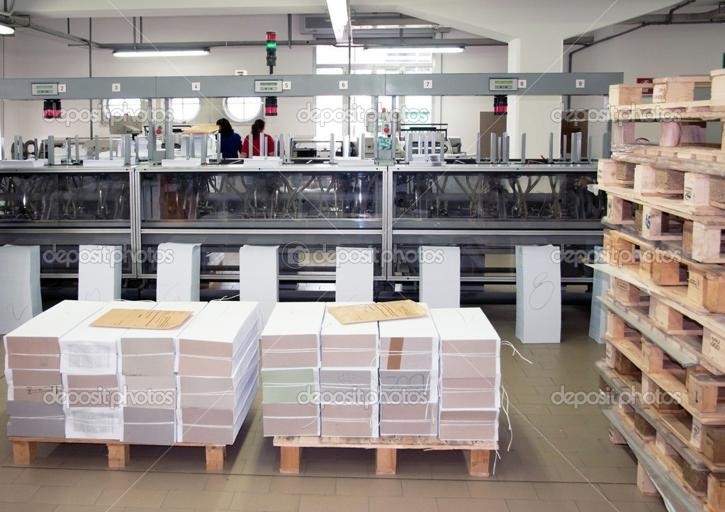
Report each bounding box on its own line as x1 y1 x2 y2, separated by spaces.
215 118 242 158
240 119 275 158
